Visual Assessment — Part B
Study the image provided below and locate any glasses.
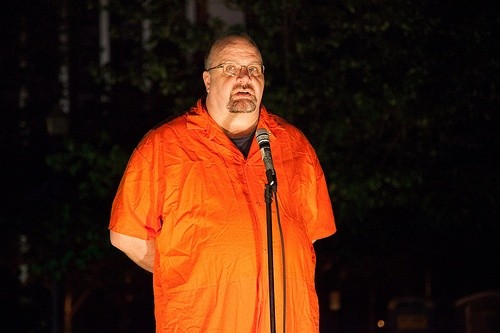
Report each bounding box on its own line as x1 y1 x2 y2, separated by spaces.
207 61 266 77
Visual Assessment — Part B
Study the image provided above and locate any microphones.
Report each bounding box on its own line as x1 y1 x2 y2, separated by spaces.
256 128 277 193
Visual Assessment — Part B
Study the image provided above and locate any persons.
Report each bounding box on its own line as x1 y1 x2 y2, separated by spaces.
108 30 337 333
